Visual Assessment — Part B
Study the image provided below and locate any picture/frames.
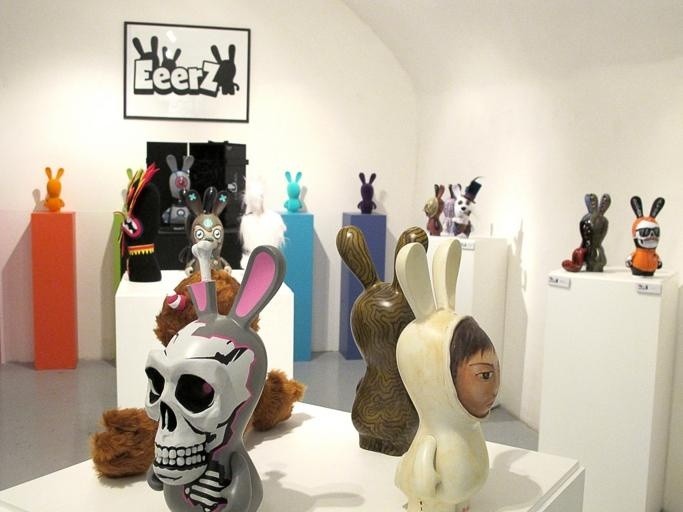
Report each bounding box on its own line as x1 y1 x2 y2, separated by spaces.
122 21 250 123
